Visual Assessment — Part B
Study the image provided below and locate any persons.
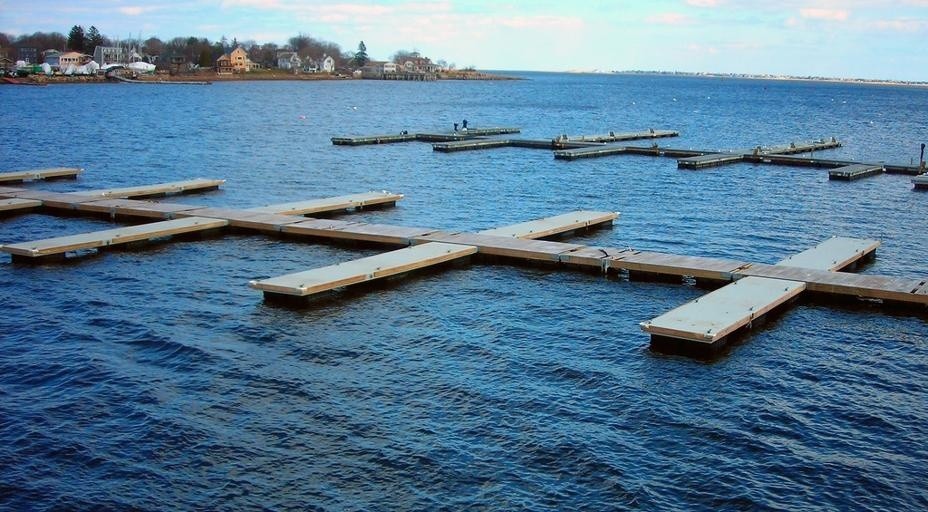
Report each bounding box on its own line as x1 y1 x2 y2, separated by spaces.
450 118 468 136
